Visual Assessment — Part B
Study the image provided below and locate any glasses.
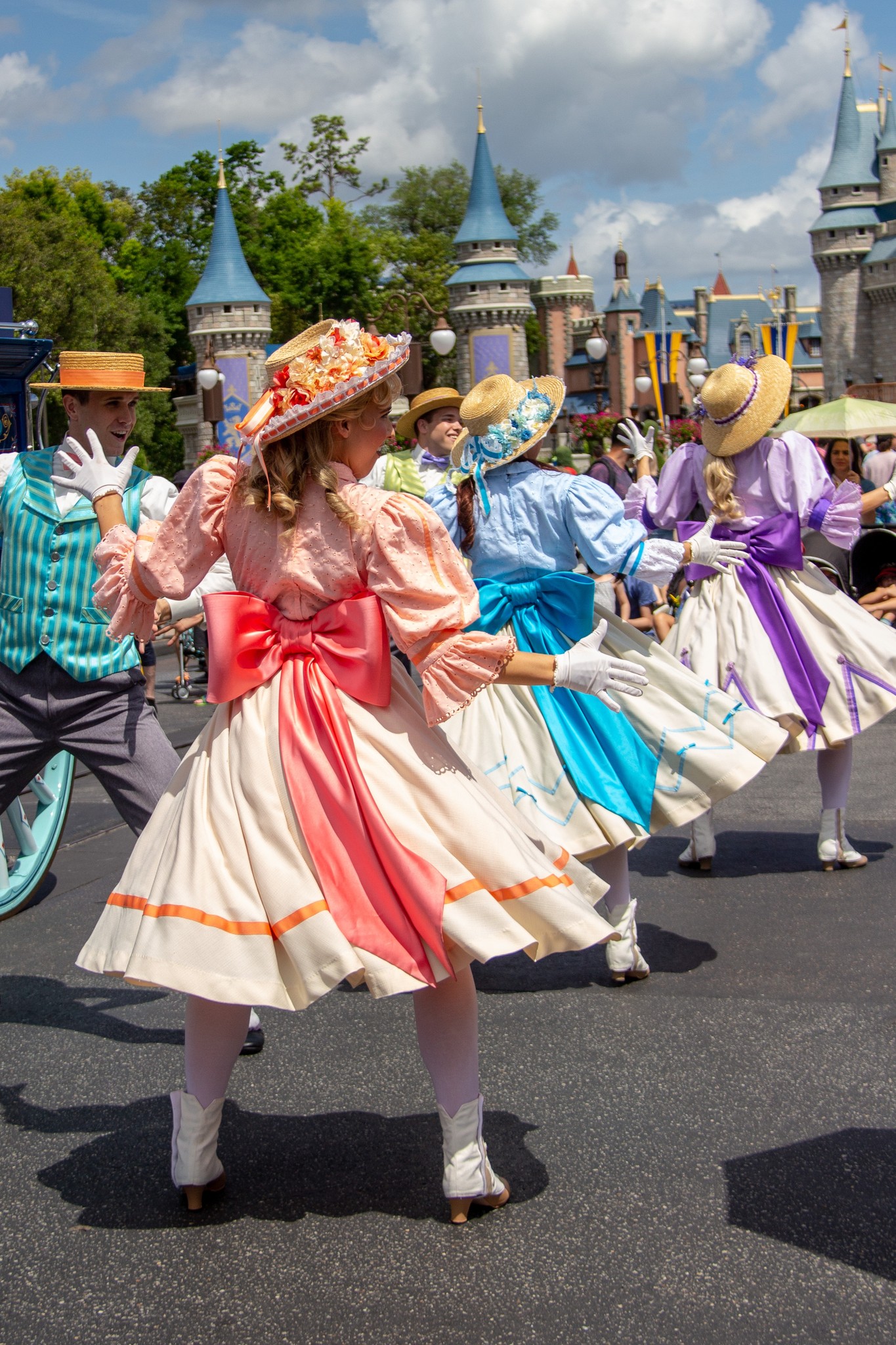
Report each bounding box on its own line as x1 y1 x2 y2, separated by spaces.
178 483 186 491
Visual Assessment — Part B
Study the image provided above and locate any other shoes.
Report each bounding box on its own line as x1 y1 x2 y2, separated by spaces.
145 696 157 712
240 1008 264 1050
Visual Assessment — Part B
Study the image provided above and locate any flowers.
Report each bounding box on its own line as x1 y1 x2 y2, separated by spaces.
272 320 399 414
487 376 556 463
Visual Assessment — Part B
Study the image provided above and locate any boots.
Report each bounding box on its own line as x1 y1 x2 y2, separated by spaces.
818 807 868 871
605 897 650 981
170 1089 226 1210
435 1092 510 1223
678 806 717 870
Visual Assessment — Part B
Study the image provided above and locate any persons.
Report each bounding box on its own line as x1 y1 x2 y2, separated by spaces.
423 374 788 982
51 317 647 1224
0 351 264 1057
857 560 896 627
358 387 466 499
809 435 896 530
129 470 239 713
572 418 696 642
618 354 896 871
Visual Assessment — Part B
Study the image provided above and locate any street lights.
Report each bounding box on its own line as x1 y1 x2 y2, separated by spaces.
365 293 457 402
201 335 227 453
632 350 709 441
585 317 614 419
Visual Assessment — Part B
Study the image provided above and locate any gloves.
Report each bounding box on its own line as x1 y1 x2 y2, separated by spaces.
550 618 649 714
50 428 139 514
883 466 896 501
617 418 655 465
682 515 750 575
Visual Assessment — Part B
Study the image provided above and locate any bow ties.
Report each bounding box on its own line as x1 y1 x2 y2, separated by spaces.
63 452 83 470
421 451 449 471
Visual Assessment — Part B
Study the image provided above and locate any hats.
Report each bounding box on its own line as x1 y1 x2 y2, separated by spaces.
818 565 838 576
222 319 412 514
173 470 193 483
877 433 896 443
700 350 792 457
876 559 896 579
395 387 465 438
29 351 173 392
446 373 567 520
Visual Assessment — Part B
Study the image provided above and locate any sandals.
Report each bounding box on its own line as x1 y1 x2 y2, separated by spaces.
192 694 214 705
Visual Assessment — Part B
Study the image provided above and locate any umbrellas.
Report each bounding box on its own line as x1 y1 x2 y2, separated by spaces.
770 394 896 471
